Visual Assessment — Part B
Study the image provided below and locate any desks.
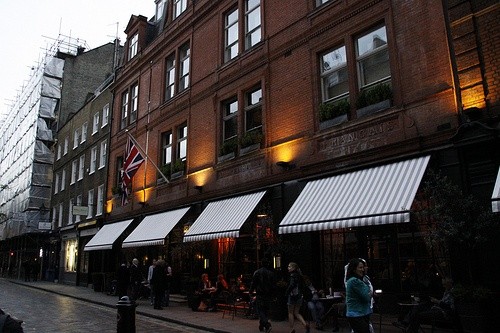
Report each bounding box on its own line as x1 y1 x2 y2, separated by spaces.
314 296 342 326
243 291 257 319
398 301 423 329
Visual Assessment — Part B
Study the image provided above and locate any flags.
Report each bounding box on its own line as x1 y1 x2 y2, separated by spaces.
121 131 146 206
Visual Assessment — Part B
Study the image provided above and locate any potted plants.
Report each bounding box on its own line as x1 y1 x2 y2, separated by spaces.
111 184 121 199
238 129 263 155
356 83 392 119
157 164 170 185
318 97 351 132
217 138 237 164
170 159 185 180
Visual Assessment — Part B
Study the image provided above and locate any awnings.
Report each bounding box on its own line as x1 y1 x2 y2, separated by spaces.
491 165 500 212
279 156 431 235
182 191 267 243
84 219 134 251
121 206 190 248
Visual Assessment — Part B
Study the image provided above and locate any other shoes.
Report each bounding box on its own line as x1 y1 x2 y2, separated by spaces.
154 306 163 310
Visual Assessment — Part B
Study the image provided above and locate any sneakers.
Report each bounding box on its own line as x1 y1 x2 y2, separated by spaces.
257 329 264 333
265 326 272 333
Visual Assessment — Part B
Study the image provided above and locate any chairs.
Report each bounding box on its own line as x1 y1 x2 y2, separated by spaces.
222 290 244 319
432 296 465 333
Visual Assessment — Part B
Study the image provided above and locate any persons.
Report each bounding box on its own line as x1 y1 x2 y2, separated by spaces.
21 256 464 333
0 307 24 333
